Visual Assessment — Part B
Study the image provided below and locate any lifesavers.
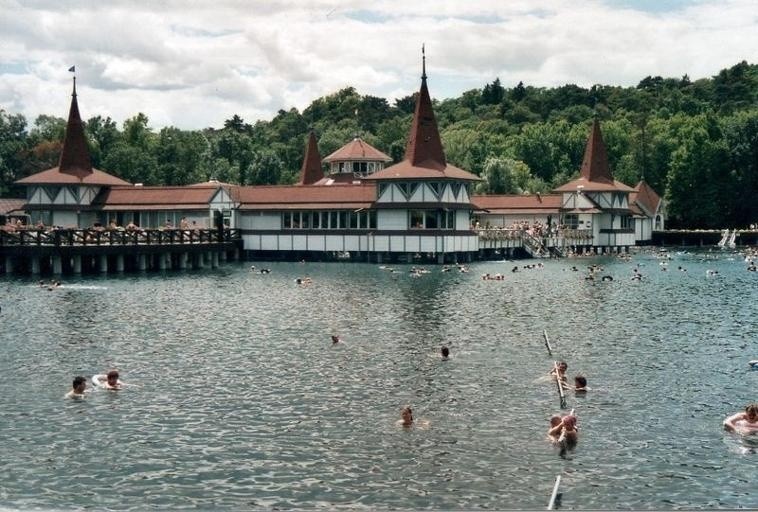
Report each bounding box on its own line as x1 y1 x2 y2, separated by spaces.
724 412 758 435
91 373 125 392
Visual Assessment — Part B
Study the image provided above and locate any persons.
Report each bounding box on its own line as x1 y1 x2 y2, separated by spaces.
85 220 147 243
251 265 255 271
102 371 136 391
161 216 205 241
551 363 588 391
38 278 64 292
331 335 343 348
295 276 313 289
548 415 577 443
724 402 756 433
299 258 305 264
64 376 90 399
406 221 757 282
397 408 415 425
441 346 449 358
0 217 65 245
